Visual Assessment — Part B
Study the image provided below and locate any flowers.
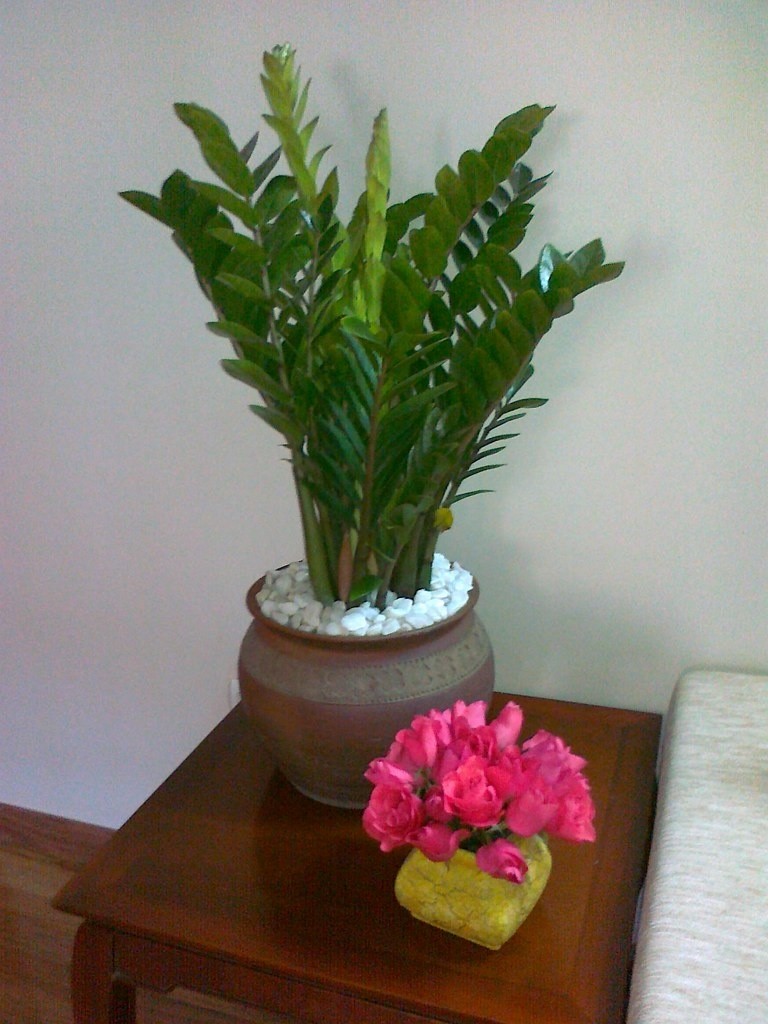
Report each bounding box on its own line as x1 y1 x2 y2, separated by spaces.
360 700 597 883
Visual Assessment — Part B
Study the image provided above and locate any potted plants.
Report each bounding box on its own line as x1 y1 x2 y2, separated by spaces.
108 44 624 808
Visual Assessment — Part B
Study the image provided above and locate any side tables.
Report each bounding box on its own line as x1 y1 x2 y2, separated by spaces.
48 692 663 1024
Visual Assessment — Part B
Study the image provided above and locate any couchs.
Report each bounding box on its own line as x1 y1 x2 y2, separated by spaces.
630 669 768 1024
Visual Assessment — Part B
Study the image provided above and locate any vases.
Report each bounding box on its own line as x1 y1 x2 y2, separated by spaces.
394 837 550 949
